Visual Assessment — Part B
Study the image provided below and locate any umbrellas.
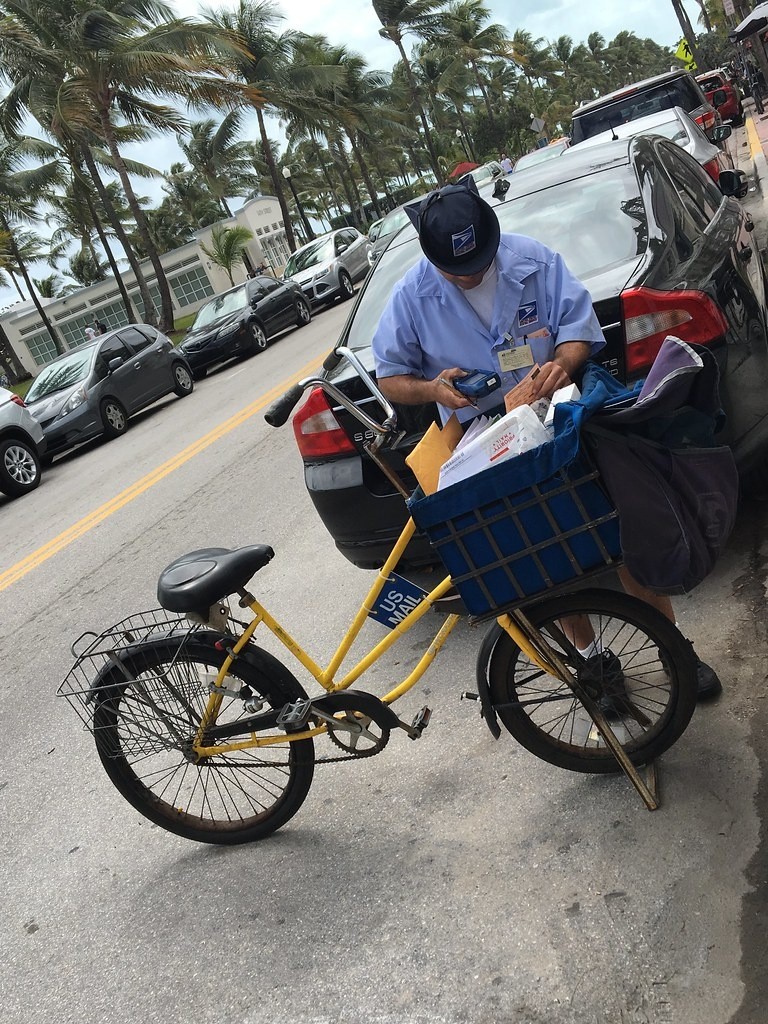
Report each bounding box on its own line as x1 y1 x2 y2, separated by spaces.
448 162 481 178
727 1 768 44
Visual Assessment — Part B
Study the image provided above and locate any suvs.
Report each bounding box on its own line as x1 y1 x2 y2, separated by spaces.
458 161 509 188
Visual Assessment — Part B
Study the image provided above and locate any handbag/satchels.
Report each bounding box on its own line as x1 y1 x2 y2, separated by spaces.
585 335 741 598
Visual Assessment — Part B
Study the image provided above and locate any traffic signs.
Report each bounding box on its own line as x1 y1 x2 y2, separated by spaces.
685 62 697 72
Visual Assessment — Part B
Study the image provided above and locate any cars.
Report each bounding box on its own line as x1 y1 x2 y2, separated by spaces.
0 388 46 499
289 131 768 570
178 275 312 382
513 137 572 172
697 73 741 125
370 192 430 259
283 227 373 307
694 67 740 104
571 64 733 160
561 106 736 187
22 323 195 464
370 218 385 243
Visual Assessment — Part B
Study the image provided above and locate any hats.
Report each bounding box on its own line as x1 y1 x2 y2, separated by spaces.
403 174 500 274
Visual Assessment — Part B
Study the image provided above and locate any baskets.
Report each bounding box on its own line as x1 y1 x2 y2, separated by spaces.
55 598 237 762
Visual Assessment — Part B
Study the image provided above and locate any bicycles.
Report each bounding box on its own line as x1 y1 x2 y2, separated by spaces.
54 343 700 846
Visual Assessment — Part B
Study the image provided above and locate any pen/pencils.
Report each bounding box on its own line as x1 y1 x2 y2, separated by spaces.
437 376 480 411
523 334 528 345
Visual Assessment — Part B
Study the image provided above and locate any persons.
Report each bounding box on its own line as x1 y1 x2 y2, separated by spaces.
370 185 725 716
500 153 514 174
381 192 399 216
2 372 14 389
424 179 452 193
95 320 107 335
84 324 97 340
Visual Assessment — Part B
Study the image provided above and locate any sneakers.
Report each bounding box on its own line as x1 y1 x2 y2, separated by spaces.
658 637 722 705
578 646 630 722
